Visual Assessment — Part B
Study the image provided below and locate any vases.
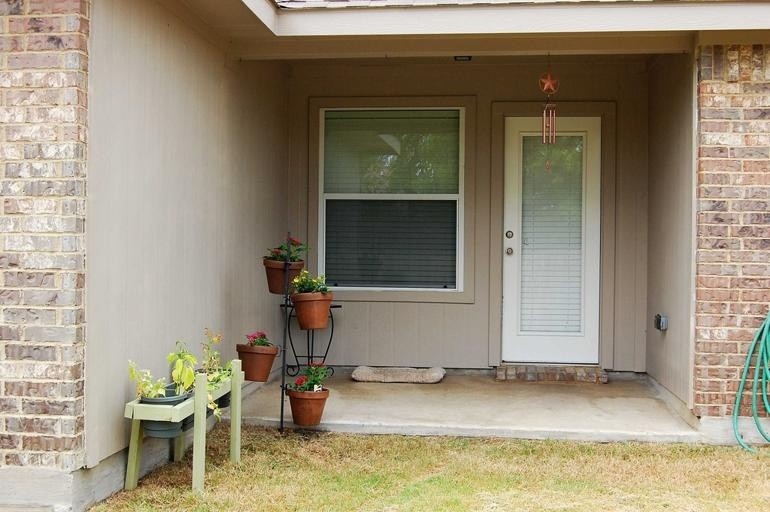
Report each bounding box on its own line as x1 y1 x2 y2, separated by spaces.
292 292 333 330
264 258 305 294
237 343 278 382
285 385 329 426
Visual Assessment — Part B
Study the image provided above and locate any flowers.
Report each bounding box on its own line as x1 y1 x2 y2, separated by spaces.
245 330 273 346
287 268 328 294
288 361 329 392
262 235 309 261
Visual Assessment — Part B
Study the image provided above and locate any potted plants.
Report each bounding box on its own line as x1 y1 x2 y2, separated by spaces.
126 328 232 439
